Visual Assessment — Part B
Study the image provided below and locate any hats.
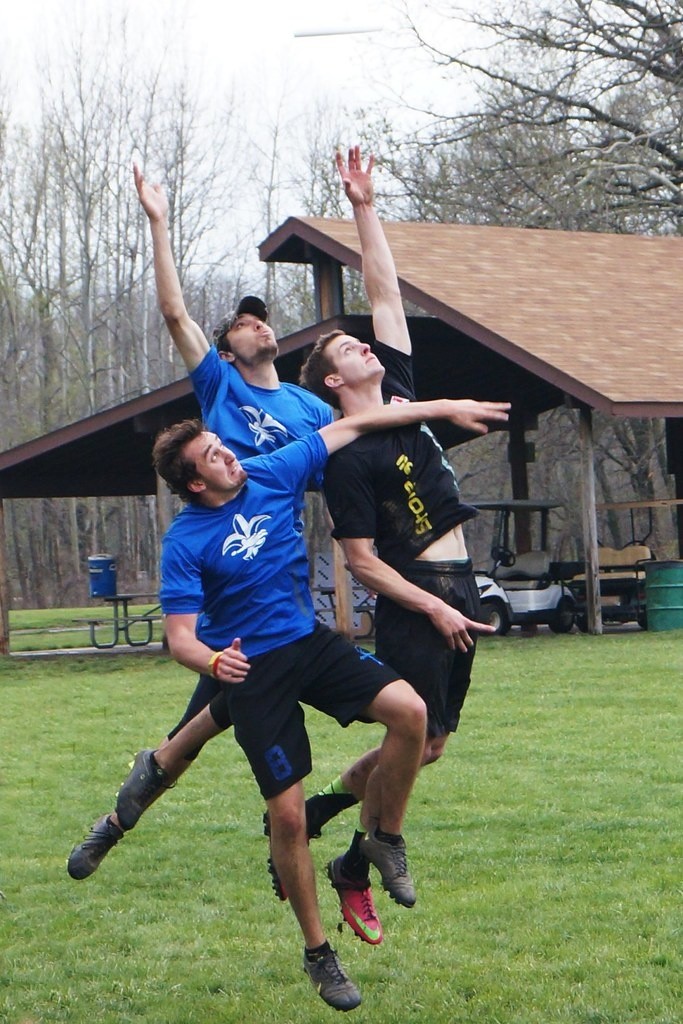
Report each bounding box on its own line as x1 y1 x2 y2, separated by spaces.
212 294 269 346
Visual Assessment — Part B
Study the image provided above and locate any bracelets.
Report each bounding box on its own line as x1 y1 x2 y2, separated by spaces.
207 652 222 679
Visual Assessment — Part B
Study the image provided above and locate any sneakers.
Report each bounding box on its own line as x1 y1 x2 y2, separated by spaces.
65 813 124 882
358 824 418 910
259 807 311 902
324 854 384 946
112 746 172 833
299 951 362 1013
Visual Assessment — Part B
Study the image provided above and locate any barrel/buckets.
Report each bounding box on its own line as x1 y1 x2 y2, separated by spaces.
643 559 682 632
87 553 115 598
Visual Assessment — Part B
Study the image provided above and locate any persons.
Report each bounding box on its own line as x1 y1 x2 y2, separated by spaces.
68 159 349 899
258 141 485 946
148 396 514 1010
